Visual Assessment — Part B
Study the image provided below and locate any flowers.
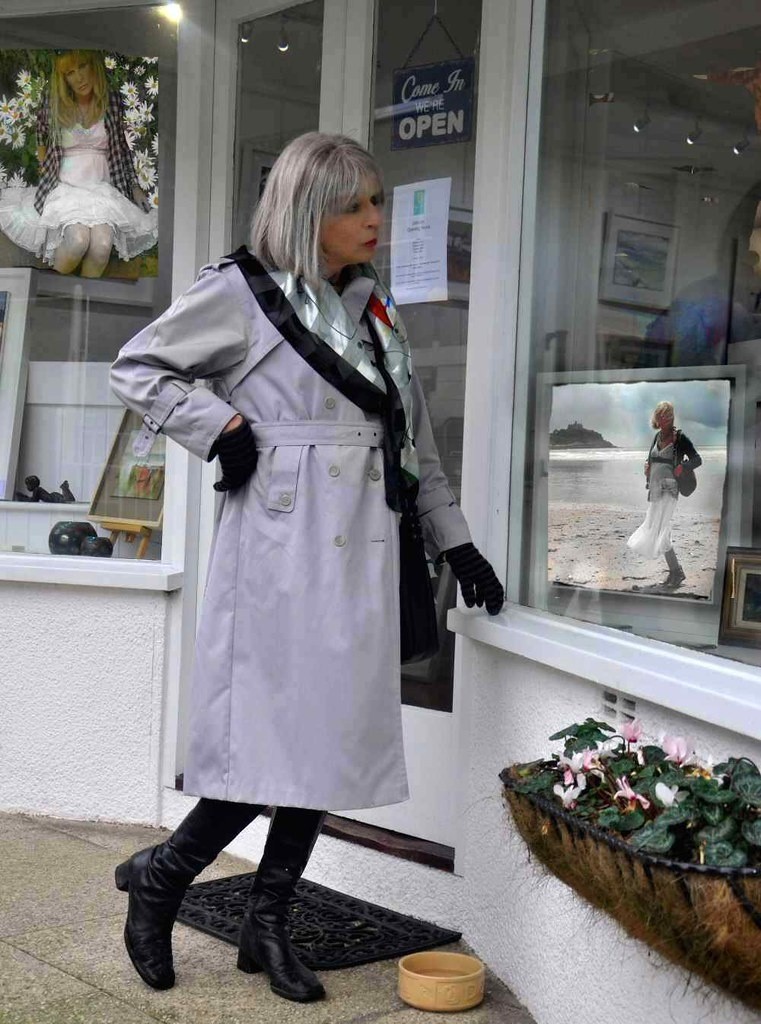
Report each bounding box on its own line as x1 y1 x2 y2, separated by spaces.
516 716 761 865
0 51 157 278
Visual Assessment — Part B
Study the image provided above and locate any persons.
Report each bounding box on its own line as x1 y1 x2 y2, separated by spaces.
14 476 75 502
627 400 701 588
0 49 158 281
114 134 503 1000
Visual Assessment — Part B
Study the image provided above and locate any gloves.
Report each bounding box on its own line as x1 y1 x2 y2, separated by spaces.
674 464 691 479
644 463 650 477
444 542 504 616
212 415 258 497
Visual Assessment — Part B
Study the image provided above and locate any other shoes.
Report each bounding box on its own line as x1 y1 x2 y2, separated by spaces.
659 565 686 590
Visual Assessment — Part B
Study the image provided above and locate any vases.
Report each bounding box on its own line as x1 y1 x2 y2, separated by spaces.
500 762 761 1011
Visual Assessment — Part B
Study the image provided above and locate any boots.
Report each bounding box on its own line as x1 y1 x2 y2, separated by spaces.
115 797 267 990
237 807 325 1004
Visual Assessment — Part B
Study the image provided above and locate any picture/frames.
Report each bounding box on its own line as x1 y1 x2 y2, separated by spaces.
719 547 760 649
600 208 681 309
448 206 472 302
239 146 285 248
0 269 36 500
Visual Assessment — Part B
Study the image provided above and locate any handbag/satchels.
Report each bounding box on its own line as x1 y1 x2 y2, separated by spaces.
399 503 441 665
673 429 697 496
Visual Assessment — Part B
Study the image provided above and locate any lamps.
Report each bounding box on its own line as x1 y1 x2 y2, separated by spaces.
734 132 750 154
634 105 651 131
241 23 251 42
274 16 289 51
686 120 703 145
